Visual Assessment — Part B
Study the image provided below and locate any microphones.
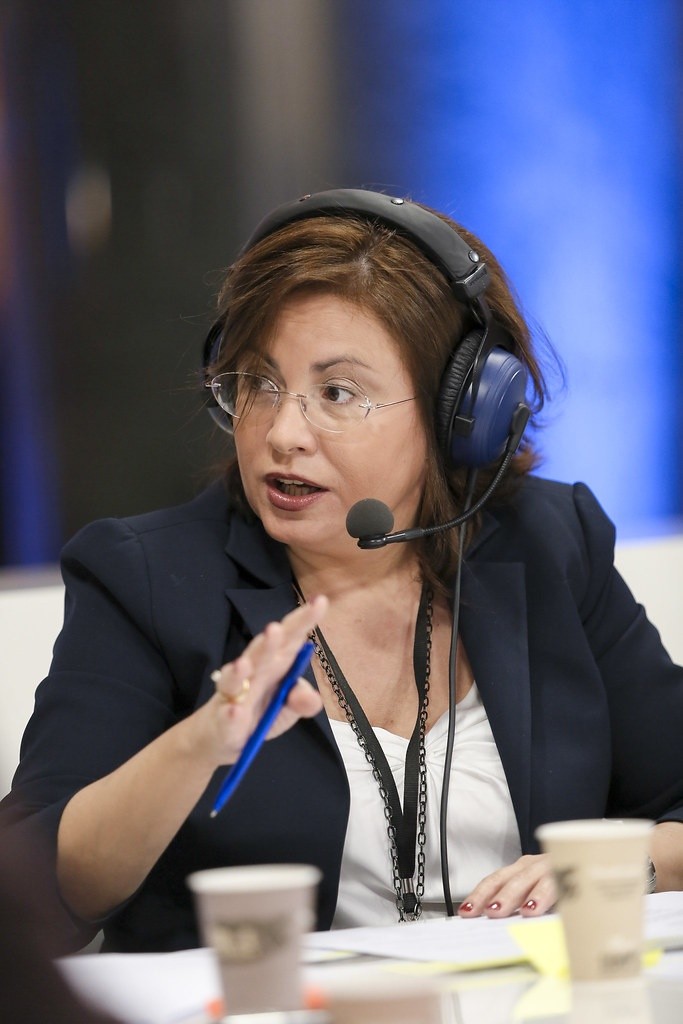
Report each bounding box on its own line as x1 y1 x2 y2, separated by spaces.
345 404 531 549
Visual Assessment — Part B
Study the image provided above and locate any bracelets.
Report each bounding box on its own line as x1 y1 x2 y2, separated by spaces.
647 858 656 894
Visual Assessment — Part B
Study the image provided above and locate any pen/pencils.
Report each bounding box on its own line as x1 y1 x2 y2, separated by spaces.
209 640 316 819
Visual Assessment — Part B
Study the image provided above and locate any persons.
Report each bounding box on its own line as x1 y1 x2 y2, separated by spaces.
1 189 683 970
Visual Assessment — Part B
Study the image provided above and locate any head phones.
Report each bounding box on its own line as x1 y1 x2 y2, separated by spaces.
200 188 532 471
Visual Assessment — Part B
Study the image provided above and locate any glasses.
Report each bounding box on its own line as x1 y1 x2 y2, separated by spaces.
204 372 417 434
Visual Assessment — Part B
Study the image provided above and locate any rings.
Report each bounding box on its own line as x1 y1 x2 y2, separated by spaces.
211 665 250 704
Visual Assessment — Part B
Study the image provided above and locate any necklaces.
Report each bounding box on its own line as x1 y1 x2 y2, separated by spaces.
290 573 434 920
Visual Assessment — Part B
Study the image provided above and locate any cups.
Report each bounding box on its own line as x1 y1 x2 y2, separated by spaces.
536 819 651 979
189 865 320 1008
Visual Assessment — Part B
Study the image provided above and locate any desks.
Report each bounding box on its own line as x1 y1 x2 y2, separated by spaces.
55 890 683 1024
